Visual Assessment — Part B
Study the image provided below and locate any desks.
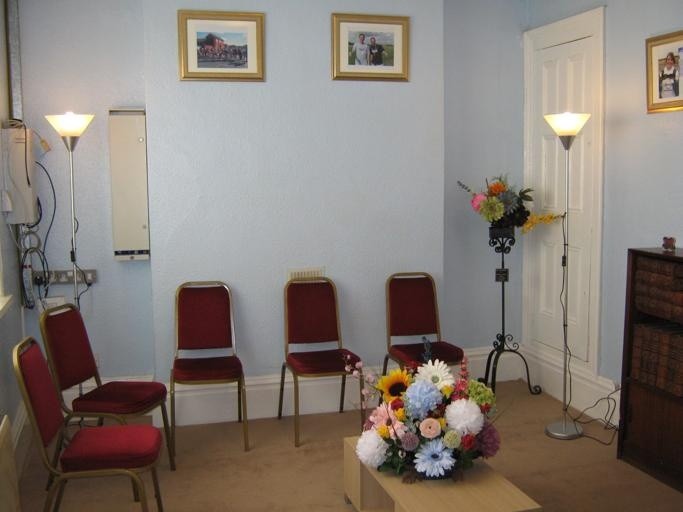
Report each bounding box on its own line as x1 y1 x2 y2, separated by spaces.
341 432 544 511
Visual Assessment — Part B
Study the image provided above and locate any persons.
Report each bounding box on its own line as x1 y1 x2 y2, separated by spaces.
348 34 370 67
197 39 245 62
369 36 387 67
657 50 680 100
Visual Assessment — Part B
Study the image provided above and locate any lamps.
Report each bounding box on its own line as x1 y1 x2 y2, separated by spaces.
543 109 593 441
45 110 95 398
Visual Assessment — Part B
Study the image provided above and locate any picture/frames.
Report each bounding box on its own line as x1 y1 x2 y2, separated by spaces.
176 9 267 83
330 11 411 82
642 25 683 116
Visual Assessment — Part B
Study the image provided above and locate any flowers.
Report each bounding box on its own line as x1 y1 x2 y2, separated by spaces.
342 353 504 485
455 173 563 234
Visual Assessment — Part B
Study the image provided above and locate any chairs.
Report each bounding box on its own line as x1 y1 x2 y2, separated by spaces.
378 270 467 408
276 274 367 449
37 303 175 489
167 278 250 456
11 332 168 511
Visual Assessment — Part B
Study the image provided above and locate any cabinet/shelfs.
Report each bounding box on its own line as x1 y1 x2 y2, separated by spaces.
615 244 683 495
